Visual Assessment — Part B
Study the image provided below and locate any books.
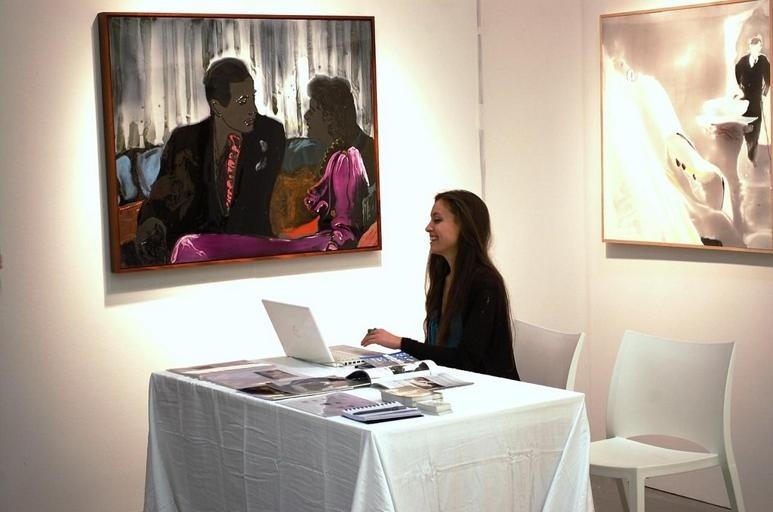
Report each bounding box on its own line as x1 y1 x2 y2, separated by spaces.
168 346 475 423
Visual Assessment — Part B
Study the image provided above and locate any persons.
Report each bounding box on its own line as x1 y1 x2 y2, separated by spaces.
359 190 519 379
731 34 770 165
132 54 286 267
170 74 370 266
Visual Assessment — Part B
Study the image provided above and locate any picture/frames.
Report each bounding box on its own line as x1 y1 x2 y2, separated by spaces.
97 13 382 275
598 0 773 256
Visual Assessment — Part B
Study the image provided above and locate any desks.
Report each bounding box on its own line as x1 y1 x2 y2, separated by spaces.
151 344 585 512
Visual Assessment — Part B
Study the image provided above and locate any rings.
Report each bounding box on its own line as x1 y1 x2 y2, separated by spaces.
367 328 375 335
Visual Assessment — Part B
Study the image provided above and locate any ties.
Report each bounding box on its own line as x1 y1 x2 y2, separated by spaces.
224 133 242 210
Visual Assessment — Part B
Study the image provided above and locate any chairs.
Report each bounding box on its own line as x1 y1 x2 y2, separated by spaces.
510 321 585 391
590 329 746 512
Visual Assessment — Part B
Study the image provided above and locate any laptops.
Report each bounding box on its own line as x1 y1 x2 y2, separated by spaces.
261 299 387 367
